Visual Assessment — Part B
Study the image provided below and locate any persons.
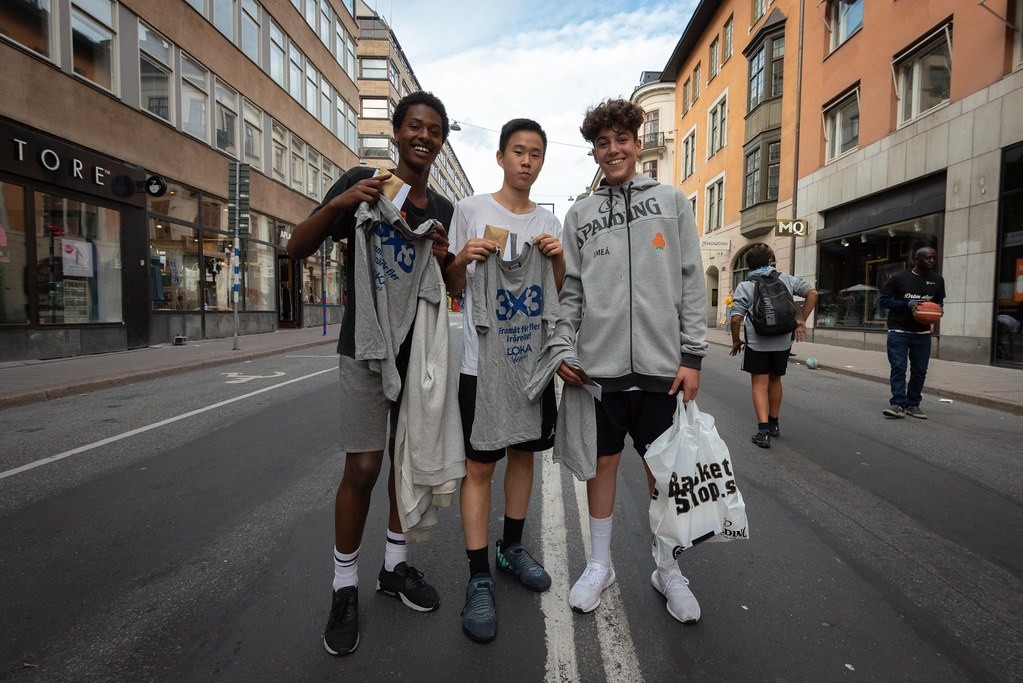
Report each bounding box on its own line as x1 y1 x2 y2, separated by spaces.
287 91 438 659
730 243 818 448
881 248 946 419
444 119 566 643
725 288 737 336
553 99 711 622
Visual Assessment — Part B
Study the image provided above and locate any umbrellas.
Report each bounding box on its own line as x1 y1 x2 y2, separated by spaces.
839 283 879 293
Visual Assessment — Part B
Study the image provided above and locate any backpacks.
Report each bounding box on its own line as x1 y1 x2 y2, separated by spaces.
742 272 799 337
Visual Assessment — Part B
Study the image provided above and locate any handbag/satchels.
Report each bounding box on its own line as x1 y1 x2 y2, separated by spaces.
644 391 750 567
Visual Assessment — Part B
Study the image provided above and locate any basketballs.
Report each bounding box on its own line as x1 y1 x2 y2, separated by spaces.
913 301 941 324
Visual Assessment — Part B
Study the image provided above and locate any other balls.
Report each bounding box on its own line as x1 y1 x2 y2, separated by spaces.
806 358 818 369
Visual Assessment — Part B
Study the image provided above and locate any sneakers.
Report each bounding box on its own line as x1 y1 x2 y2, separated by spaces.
881 404 929 419
752 425 782 449
495 541 552 594
650 564 702 625
324 585 361 657
375 560 441 613
460 570 497 646
568 558 617 614
